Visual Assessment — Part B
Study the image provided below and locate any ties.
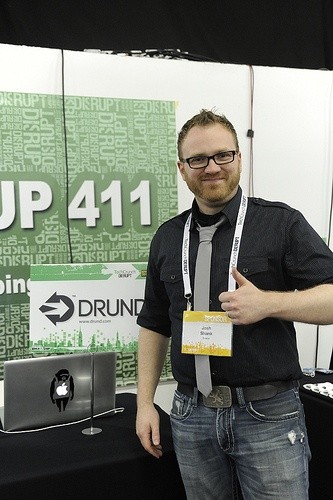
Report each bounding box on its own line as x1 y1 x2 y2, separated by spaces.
194 216 226 397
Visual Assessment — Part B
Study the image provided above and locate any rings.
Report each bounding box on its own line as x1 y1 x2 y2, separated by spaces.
227 312 231 318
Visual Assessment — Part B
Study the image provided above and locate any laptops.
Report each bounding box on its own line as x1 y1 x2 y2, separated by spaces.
0 351 117 434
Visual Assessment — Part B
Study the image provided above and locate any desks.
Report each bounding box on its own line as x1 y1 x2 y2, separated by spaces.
1 392 183 500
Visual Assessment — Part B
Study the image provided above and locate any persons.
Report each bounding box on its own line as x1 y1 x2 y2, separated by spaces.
133 107 333 500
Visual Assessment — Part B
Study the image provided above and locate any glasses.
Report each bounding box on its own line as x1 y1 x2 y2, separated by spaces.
180 149 238 169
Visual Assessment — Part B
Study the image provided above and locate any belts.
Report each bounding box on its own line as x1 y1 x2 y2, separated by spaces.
176 379 300 409
